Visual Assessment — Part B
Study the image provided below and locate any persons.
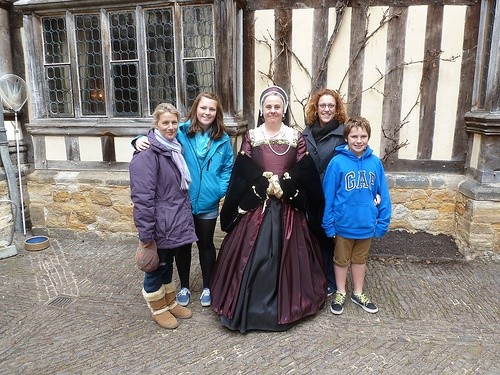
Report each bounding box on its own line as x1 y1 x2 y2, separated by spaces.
131 92 234 307
302 88 350 296
323 116 391 316
129 103 192 329
210 86 328 332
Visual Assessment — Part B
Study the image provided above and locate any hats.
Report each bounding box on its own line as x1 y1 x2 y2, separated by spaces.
135 238 158 272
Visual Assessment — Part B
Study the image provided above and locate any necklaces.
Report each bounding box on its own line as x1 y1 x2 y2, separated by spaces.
262 122 290 155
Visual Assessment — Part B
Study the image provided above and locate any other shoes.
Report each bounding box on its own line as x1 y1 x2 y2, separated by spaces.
327 286 335 296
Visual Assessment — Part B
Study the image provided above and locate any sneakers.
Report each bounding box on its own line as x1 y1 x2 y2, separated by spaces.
330 291 347 313
351 292 378 313
199 288 211 306
175 288 190 305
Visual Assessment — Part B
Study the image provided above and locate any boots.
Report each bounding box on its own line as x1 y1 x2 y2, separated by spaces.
165 278 192 318
142 283 178 329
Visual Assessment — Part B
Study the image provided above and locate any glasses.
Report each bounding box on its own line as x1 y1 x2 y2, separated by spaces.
319 103 335 110
